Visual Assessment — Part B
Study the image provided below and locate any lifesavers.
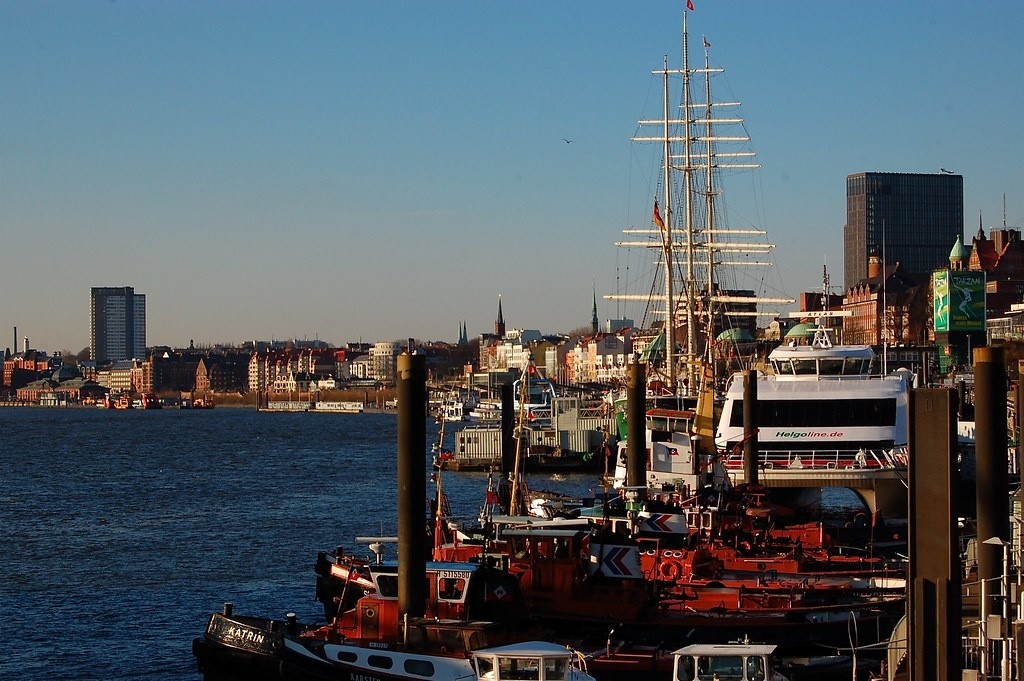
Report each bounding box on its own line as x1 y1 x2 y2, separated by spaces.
659 561 678 580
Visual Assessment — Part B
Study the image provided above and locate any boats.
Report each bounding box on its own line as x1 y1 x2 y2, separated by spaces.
191 3 970 681
